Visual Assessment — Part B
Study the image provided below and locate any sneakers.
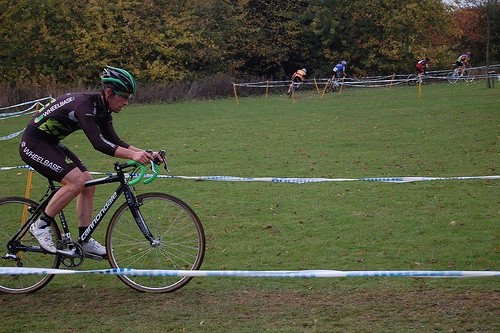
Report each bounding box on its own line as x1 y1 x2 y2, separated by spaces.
29 223 58 255
76 236 114 256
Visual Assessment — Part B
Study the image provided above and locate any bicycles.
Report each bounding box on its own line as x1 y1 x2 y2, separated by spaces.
446 67 477 84
409 69 429 83
0 149 205 294
288 80 300 97
325 76 343 90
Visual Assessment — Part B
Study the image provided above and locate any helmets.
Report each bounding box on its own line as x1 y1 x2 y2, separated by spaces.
101 64 138 96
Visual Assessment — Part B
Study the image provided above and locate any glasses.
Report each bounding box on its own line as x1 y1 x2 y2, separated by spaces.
113 89 132 100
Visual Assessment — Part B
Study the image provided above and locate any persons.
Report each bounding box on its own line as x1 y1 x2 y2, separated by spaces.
19 65 168 254
416 57 431 76
329 61 348 90
452 51 474 70
288 68 306 95
